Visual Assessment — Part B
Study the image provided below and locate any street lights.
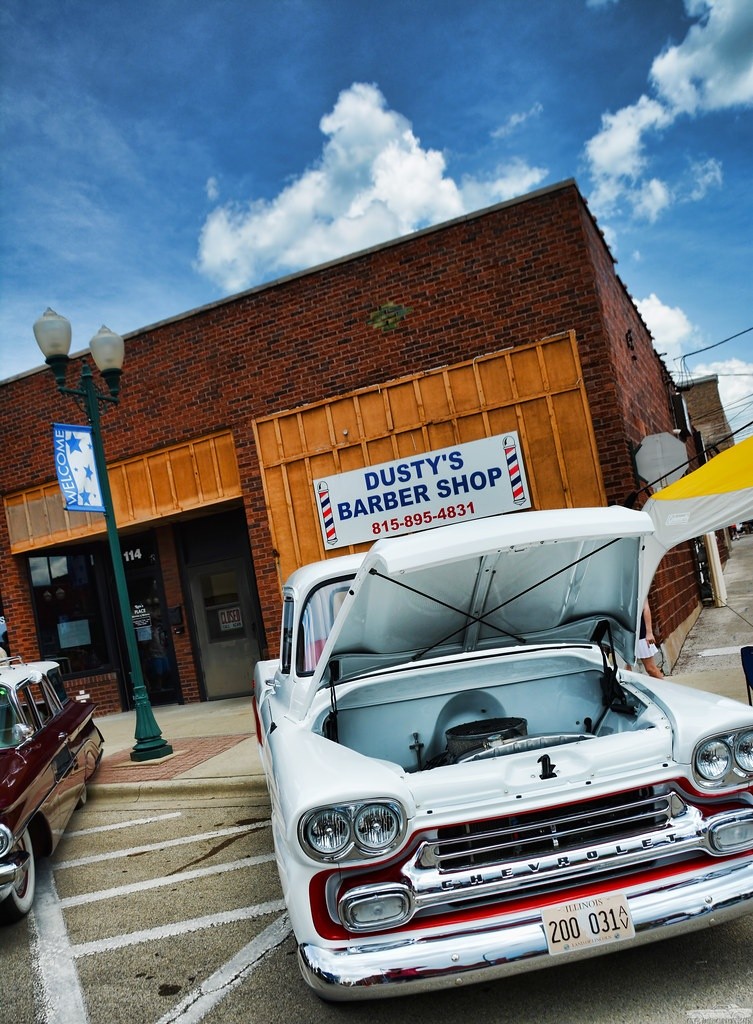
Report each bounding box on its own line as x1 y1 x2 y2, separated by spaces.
33 307 174 763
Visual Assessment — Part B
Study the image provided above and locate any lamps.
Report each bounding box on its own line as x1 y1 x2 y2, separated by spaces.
56 588 65 600
44 591 51 602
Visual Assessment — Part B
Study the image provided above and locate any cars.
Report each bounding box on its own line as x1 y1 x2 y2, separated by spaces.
251 506 753 1003
0 656 106 925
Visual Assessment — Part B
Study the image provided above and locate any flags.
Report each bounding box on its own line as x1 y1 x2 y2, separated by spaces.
50 420 106 513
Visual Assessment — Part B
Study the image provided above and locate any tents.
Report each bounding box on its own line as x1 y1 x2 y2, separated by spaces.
634 435 753 661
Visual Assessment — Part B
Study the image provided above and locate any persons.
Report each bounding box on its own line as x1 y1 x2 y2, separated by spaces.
147 591 174 668
624 600 663 679
138 639 163 692
729 521 753 540
